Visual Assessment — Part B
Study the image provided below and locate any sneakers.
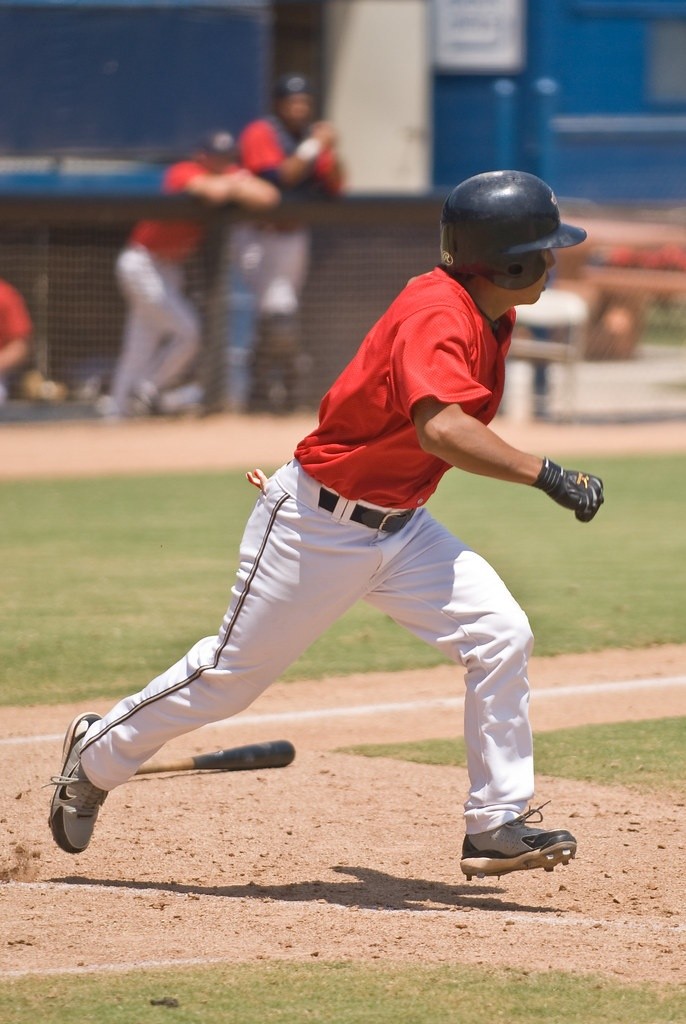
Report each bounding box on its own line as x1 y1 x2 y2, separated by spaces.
459 817 580 881
48 711 108 855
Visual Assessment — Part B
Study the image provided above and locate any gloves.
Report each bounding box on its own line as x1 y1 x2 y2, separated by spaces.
532 456 605 522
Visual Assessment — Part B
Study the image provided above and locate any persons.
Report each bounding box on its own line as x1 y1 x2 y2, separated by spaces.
46 169 605 879
0 73 349 419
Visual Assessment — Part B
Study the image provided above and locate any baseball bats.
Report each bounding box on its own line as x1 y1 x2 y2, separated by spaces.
129 739 296 780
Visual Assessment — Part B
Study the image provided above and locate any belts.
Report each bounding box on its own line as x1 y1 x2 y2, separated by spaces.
318 490 418 536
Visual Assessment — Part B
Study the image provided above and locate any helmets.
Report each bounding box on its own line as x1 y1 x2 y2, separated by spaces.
440 170 590 290
